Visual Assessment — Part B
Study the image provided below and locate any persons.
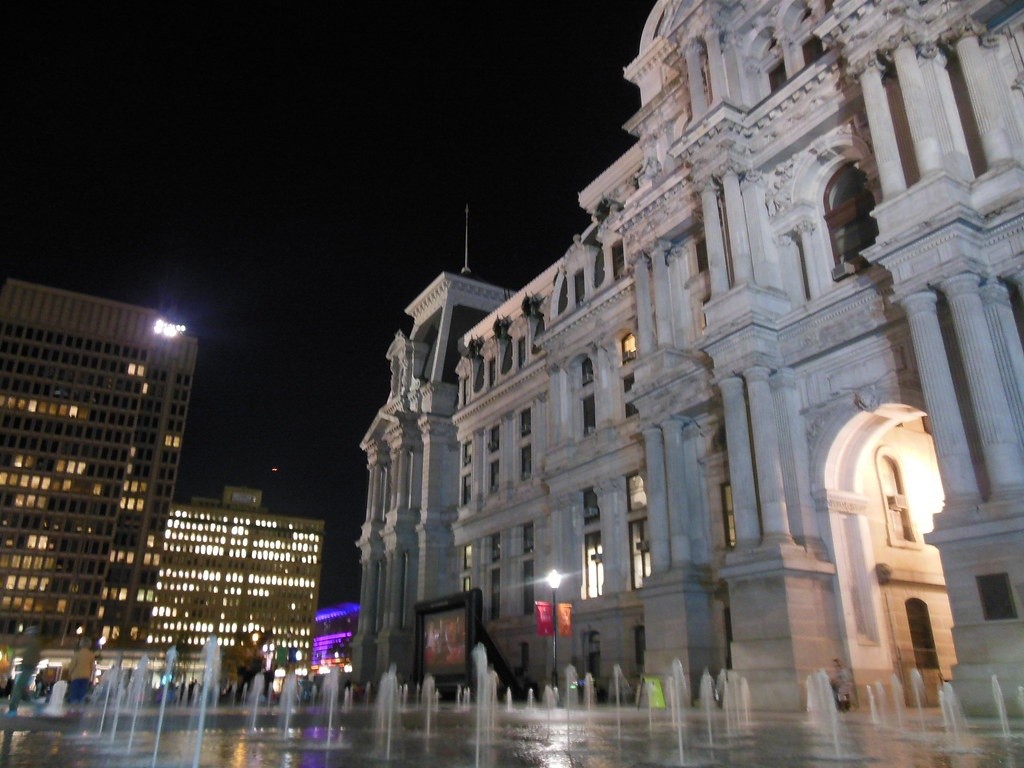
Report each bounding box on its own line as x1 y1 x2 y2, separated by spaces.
830 659 851 713
0 620 276 719
438 617 464 664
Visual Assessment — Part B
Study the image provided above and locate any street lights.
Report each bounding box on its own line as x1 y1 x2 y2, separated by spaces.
116 304 191 651
545 569 566 688
